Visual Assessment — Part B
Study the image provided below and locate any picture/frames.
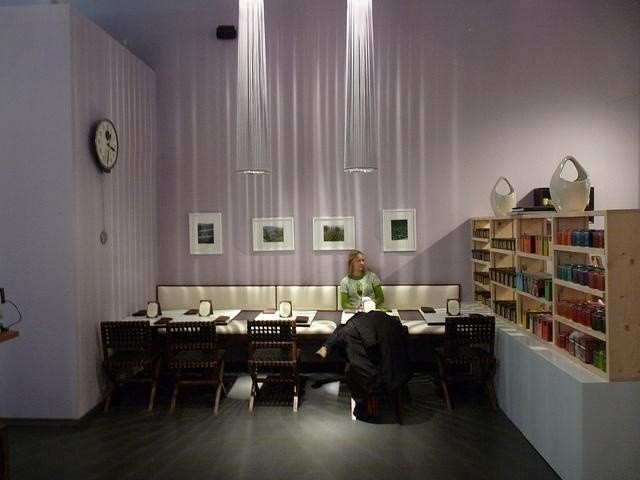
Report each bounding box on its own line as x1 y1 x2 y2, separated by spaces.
251 217 296 253
187 212 224 256
382 208 418 252
312 215 356 251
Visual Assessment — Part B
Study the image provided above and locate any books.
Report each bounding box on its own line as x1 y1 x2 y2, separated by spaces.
505 205 557 217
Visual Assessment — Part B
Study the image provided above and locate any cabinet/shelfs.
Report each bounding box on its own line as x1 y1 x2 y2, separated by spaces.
470 208 640 480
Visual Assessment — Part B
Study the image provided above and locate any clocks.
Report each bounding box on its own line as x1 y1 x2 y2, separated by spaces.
88 117 120 174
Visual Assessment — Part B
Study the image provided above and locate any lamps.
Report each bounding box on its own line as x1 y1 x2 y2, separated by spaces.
234 0 276 177
0 287 22 333
343 1 379 174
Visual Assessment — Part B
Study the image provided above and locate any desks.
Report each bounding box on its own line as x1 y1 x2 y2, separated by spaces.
0 328 20 343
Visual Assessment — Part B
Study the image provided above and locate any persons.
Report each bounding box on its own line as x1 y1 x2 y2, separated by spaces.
313 249 384 363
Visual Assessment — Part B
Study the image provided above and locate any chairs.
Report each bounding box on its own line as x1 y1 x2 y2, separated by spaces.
444 316 499 414
164 320 227 414
100 318 160 415
339 312 410 416
244 318 301 413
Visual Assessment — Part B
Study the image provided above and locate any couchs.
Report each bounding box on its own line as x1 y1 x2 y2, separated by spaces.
112 283 462 361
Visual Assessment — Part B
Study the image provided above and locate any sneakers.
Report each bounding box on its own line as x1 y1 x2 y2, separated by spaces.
316 346 328 359
